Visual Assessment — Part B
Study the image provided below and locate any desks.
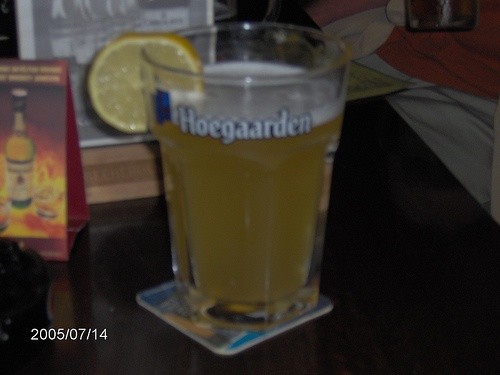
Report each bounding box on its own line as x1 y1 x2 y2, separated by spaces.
0 0 500 375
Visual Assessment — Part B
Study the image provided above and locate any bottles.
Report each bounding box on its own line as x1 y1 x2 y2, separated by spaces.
6 91 35 209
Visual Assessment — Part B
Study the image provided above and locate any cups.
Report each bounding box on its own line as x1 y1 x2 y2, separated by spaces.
140 22 352 330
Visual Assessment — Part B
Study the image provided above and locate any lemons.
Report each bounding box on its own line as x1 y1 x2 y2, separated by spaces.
86 31 207 134
